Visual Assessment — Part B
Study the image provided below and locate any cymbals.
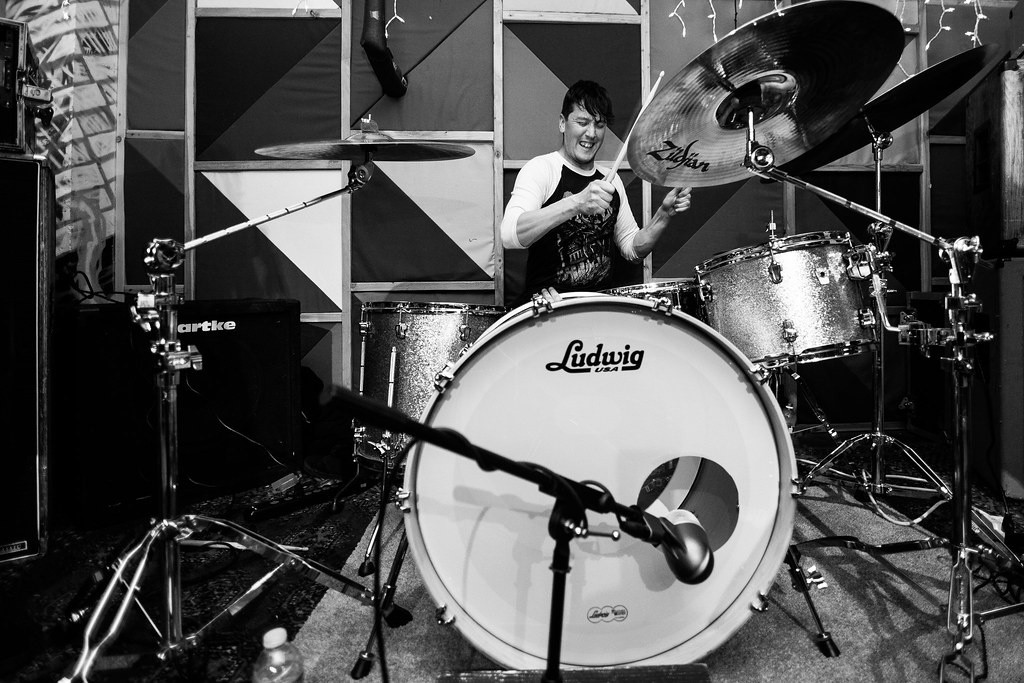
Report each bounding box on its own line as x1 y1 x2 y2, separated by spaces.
764 41 1000 186
253 130 477 162
625 2 904 188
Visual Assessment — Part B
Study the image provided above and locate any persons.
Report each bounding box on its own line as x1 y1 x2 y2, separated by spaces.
500 80 692 295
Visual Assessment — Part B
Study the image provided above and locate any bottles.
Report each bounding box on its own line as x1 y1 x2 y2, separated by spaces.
253 627 305 683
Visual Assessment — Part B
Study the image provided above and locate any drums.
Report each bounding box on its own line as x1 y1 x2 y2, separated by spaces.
612 278 711 330
398 291 799 668
696 228 875 369
347 298 510 475
